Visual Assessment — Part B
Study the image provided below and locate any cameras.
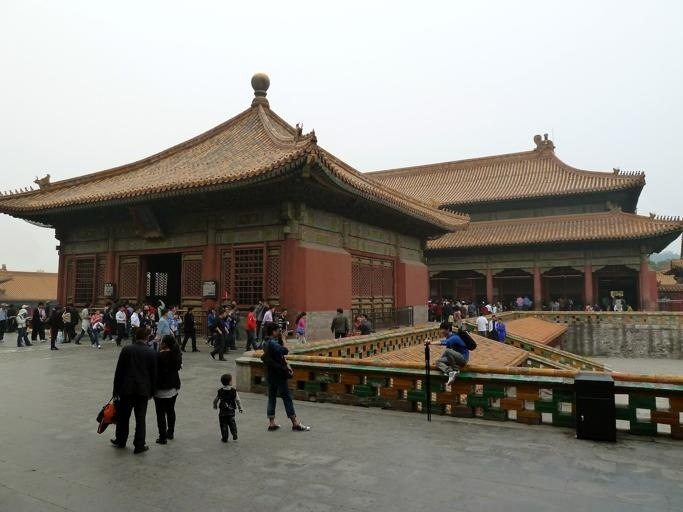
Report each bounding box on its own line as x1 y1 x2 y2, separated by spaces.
280 321 294 336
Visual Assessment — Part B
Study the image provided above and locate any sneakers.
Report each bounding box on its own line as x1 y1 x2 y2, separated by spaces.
155 435 167 445
443 370 461 386
233 433 238 440
210 351 227 363
133 445 149 455
268 423 281 432
0 337 52 347
221 437 228 443
292 422 310 432
205 339 214 349
60 337 122 349
180 346 200 354
166 432 174 440
109 438 127 448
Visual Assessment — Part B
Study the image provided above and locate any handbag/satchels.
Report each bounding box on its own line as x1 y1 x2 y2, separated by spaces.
95 396 117 434
270 357 295 378
296 327 305 335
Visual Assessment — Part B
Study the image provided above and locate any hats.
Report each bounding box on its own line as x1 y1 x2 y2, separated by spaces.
0 302 31 311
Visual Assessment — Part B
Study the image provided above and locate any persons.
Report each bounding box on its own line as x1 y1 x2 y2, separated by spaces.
111 329 157 452
331 308 374 338
213 373 243 442
152 335 182 444
425 323 469 386
262 322 310 432
428 295 634 343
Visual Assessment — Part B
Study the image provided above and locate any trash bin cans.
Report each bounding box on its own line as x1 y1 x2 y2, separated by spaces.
572 373 618 443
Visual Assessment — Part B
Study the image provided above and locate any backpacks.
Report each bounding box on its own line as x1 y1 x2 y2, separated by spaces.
456 328 477 351
